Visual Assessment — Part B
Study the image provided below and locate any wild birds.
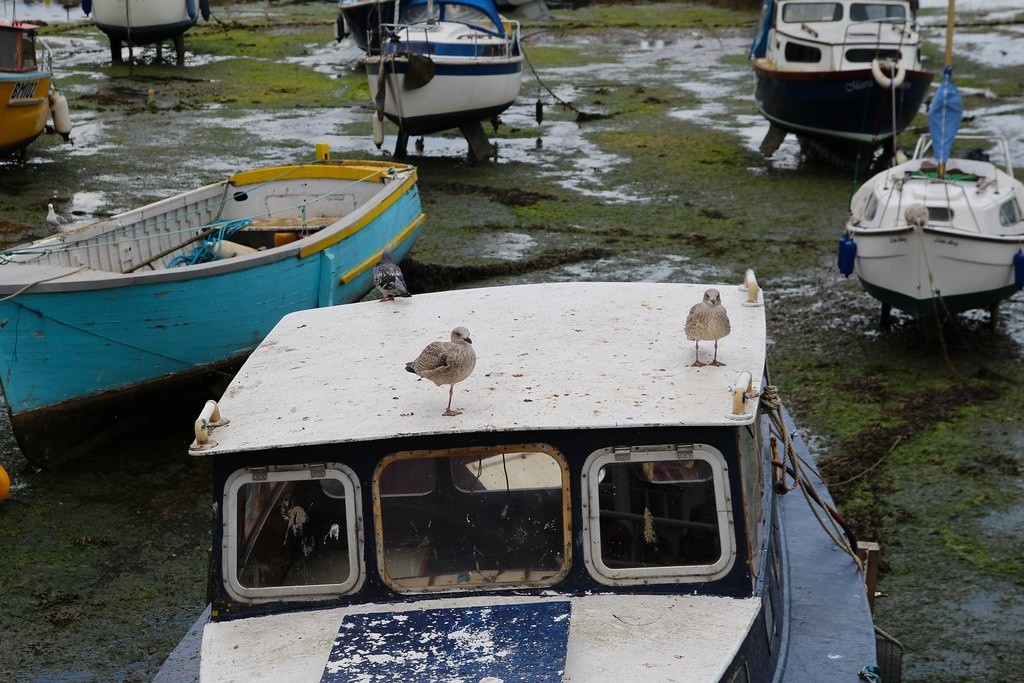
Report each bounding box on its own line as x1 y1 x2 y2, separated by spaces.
374 248 413 302
408 327 474 417
46 203 67 232
684 291 734 367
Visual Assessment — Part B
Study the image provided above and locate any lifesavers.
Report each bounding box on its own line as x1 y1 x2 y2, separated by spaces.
483 13 512 36
872 56 906 89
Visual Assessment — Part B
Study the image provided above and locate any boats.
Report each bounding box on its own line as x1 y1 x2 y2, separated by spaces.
82 0 198 46
0 141 429 471
845 134 1024 323
333 1 524 137
151 264 878 683
751 0 934 144
0 21 55 149
